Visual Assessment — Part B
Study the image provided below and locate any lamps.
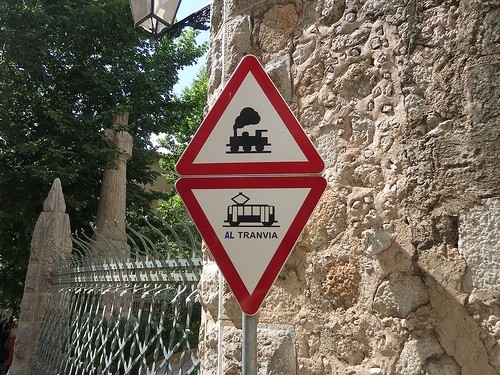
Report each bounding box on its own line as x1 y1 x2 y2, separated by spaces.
129 0 182 35
